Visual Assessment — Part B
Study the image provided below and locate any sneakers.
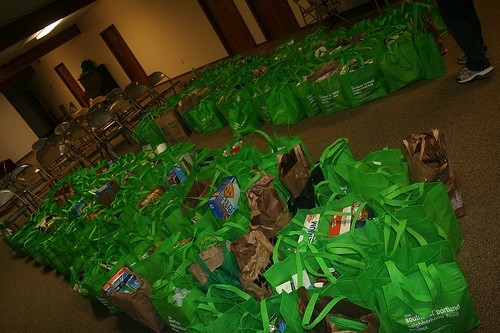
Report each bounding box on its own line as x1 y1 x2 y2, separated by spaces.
455 51 490 64
457 64 495 84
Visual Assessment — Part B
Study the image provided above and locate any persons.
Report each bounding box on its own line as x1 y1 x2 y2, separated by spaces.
435 0 494 84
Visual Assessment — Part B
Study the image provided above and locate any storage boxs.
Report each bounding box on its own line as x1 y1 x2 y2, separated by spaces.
295 214 321 244
329 204 376 237
207 176 241 219
166 152 193 186
89 175 119 209
106 271 141 296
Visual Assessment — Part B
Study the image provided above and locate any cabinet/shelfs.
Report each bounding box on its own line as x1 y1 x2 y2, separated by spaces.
77 64 119 107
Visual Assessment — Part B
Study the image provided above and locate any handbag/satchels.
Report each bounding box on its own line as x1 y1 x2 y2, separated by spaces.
131 1 447 147
10 131 481 333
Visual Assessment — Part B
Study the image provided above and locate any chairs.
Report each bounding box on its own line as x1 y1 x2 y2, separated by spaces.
57 105 70 123
293 0 346 34
1 71 183 241
